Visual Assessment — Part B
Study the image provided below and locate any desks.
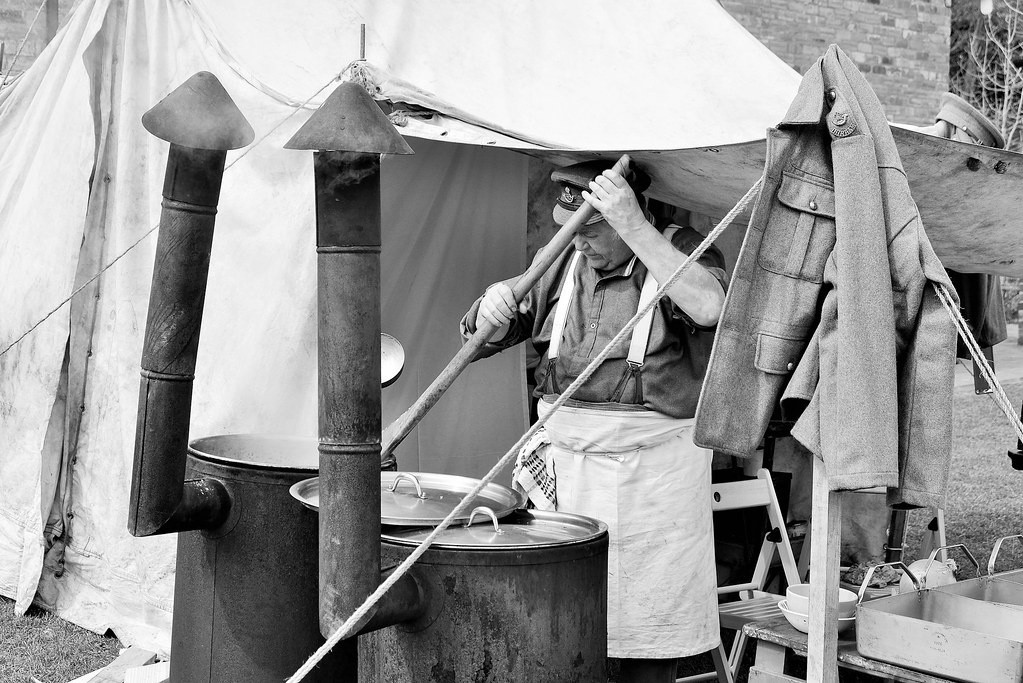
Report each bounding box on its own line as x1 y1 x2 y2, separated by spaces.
742 608 961 683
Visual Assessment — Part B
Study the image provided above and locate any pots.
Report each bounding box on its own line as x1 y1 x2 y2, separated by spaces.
169 433 397 683
357 509 609 683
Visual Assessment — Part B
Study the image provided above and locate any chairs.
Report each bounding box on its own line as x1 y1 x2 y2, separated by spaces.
665 468 803 683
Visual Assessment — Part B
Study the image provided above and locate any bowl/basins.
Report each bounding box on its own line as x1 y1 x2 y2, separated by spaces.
778 600 856 633
787 584 858 618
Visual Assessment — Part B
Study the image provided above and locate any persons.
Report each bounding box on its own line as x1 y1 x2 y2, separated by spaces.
459 156 729 683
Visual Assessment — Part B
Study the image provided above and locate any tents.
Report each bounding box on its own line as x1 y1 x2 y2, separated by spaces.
0 0 1023 660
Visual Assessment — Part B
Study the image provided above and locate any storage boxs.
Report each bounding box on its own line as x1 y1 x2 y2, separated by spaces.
855 534 1023 683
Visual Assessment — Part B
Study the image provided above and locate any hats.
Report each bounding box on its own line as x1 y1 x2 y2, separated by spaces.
935 92 1005 151
551 160 652 226
